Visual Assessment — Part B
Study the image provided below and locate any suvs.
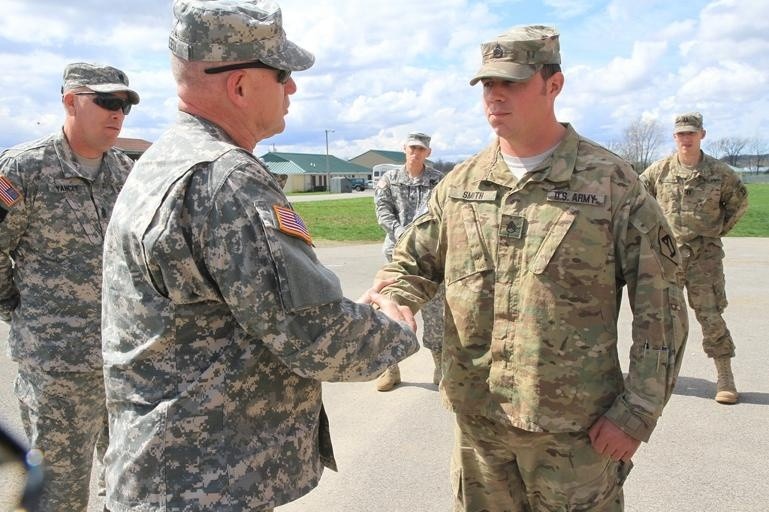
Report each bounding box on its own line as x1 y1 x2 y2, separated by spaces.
348 178 369 191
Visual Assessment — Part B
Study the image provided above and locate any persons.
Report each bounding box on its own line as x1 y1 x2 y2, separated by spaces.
370 24 689 512
0 61 139 512
639 112 749 403
101 0 421 512
373 132 445 392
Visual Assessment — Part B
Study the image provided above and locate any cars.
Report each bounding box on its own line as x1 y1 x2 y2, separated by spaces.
367 179 372 190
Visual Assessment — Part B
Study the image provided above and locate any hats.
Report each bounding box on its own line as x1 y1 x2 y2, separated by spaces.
469 25 562 87
674 112 703 134
168 0 316 71
61 63 140 105
406 131 431 150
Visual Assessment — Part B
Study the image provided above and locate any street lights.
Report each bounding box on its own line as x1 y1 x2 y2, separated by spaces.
322 128 335 192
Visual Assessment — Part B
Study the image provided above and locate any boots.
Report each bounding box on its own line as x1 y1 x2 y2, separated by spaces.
432 351 443 385
714 357 739 404
378 365 400 391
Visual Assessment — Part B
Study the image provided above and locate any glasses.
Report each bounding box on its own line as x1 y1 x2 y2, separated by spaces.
75 92 132 115
204 62 291 83
